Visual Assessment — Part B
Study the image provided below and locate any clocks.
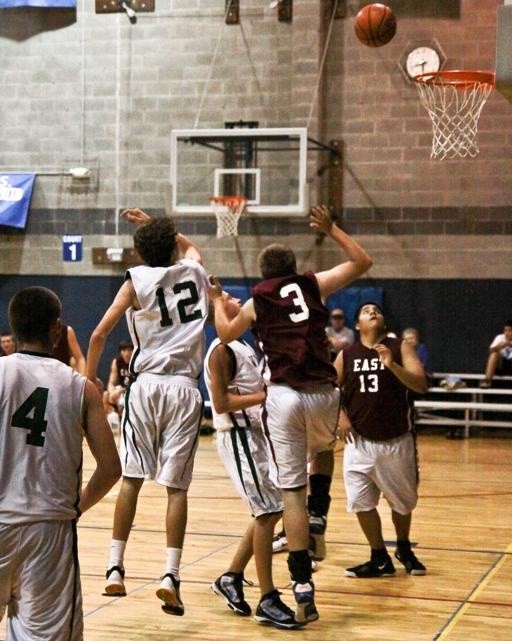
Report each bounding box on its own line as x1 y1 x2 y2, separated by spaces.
399 47 445 88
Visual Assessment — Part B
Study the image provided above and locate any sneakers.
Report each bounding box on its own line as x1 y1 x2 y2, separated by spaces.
210 571 252 615
294 575 320 624
272 529 290 553
156 573 185 616
345 557 397 579
309 512 326 562
103 568 126 598
480 379 492 387
395 544 428 576
254 591 307 631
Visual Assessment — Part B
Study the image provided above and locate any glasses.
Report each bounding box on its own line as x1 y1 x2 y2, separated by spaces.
331 314 346 320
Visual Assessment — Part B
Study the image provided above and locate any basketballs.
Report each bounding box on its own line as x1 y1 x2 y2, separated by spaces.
354 2 396 47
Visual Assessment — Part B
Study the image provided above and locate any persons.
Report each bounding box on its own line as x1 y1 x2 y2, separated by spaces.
402 327 433 398
0 331 17 356
85 208 207 617
206 204 373 624
0 285 122 641
268 443 290 552
102 338 134 417
204 291 306 631
333 301 428 580
53 322 86 377
324 306 355 362
480 322 512 387
